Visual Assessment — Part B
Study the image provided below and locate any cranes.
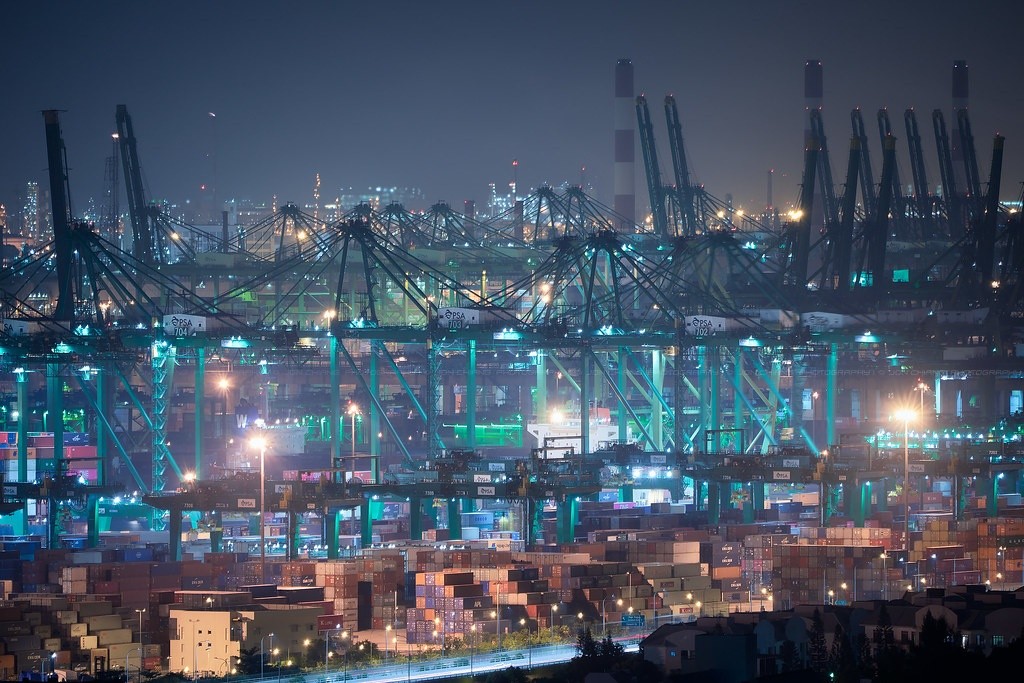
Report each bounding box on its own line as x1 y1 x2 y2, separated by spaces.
1 93 1024 560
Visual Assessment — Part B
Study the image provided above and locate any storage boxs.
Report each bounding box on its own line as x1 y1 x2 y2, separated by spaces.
0 432 1024 680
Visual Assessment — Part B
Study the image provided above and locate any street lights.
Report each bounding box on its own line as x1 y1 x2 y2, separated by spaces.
260 633 274 679
350 404 359 478
812 391 818 440
134 607 147 644
250 437 267 585
901 407 912 550
125 647 139 683
881 553 888 601
919 382 926 433
384 624 392 659
550 605 558 641
521 618 532 667
